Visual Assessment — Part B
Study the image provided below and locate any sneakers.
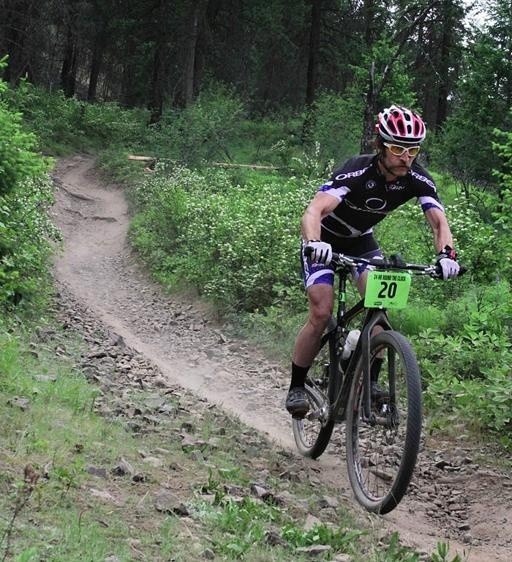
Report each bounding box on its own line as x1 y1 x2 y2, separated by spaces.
362 382 390 406
286 387 309 415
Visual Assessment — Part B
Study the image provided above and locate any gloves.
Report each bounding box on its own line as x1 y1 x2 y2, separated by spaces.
307 240 332 266
438 258 460 281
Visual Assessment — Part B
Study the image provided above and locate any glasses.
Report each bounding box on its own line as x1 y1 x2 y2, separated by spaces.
382 141 420 157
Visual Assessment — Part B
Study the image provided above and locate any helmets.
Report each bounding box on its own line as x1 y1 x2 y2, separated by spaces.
375 103 427 144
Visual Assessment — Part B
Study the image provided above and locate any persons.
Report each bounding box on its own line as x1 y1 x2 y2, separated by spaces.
285 103 461 415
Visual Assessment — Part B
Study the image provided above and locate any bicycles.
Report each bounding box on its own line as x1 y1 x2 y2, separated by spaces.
291 245 467 515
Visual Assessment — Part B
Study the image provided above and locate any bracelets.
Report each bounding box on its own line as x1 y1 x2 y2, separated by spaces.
436 243 457 263
307 239 320 244
